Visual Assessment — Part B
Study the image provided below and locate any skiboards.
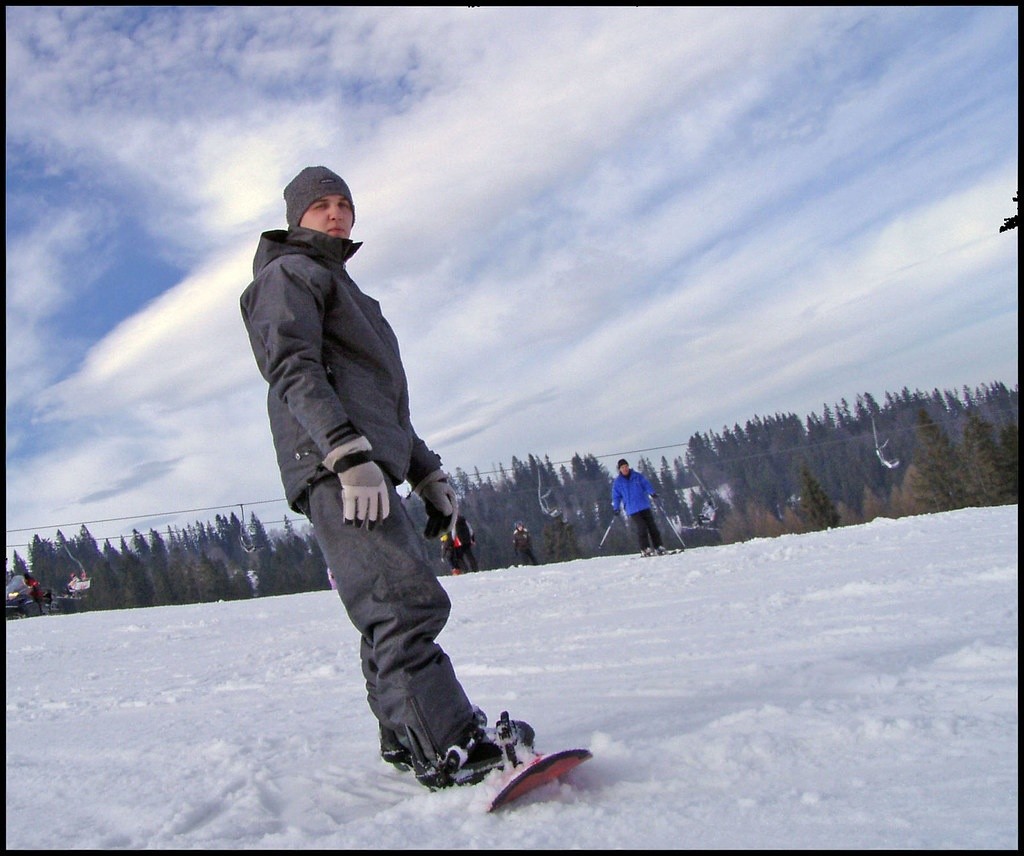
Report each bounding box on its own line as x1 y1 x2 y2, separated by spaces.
629 548 685 560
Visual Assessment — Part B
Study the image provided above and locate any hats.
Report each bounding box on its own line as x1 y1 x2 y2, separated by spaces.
283 166 355 227
618 459 629 469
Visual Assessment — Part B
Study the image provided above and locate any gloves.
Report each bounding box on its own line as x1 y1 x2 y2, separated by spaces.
613 509 621 516
651 491 658 498
413 468 459 543
321 436 391 532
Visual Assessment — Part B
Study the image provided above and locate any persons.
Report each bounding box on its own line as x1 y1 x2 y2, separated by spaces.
22 571 47 616
65 572 88 600
610 458 666 556
509 521 541 569
436 509 478 576
239 164 539 793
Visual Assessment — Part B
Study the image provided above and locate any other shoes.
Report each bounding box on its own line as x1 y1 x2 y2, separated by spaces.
656 546 668 556
640 547 654 558
415 708 536 792
381 748 415 772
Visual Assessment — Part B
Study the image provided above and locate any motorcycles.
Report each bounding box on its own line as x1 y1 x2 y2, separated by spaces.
6 575 53 620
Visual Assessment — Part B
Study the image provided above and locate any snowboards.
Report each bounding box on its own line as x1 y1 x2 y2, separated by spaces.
487 746 592 814
